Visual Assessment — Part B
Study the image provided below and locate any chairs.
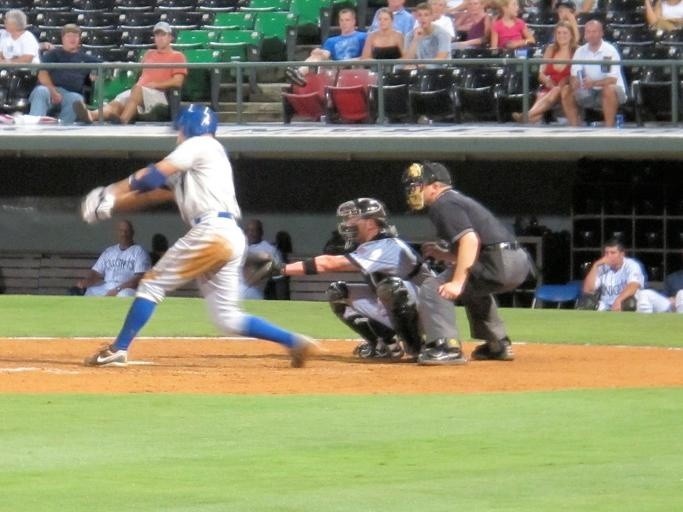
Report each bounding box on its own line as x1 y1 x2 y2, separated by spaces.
530 284 580 312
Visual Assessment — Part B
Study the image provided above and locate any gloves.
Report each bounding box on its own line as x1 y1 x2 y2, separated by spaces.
81 186 114 226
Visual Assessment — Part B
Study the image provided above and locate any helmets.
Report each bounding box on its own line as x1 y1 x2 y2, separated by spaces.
174 104 217 137
337 198 388 227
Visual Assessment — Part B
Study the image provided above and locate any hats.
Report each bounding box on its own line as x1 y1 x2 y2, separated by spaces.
401 161 454 186
153 21 171 34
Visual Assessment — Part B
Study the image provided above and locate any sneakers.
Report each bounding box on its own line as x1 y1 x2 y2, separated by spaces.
353 341 467 366
471 339 515 361
84 345 128 368
285 67 307 87
290 334 318 367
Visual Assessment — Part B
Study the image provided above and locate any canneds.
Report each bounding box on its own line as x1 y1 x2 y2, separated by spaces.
577 69 585 83
616 115 623 128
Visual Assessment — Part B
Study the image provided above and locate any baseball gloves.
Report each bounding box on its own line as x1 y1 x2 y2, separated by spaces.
241 250 281 288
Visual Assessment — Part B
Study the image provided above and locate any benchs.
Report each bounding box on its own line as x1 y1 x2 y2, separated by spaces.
35 253 103 296
286 266 380 301
0 250 42 296
149 249 265 298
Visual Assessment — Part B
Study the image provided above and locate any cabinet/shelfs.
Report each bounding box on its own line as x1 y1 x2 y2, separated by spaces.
563 179 682 287
496 232 543 307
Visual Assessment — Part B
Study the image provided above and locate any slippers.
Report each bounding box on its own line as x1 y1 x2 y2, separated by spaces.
72 100 93 123
108 113 123 124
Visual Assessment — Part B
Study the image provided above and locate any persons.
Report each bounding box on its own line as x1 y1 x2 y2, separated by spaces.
77 215 294 301
279 198 438 359
71 20 189 123
0 9 40 131
78 102 320 370
29 23 99 126
405 160 531 365
583 237 683 317
285 0 683 137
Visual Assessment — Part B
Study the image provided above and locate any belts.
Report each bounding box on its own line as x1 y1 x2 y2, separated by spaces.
482 240 521 252
